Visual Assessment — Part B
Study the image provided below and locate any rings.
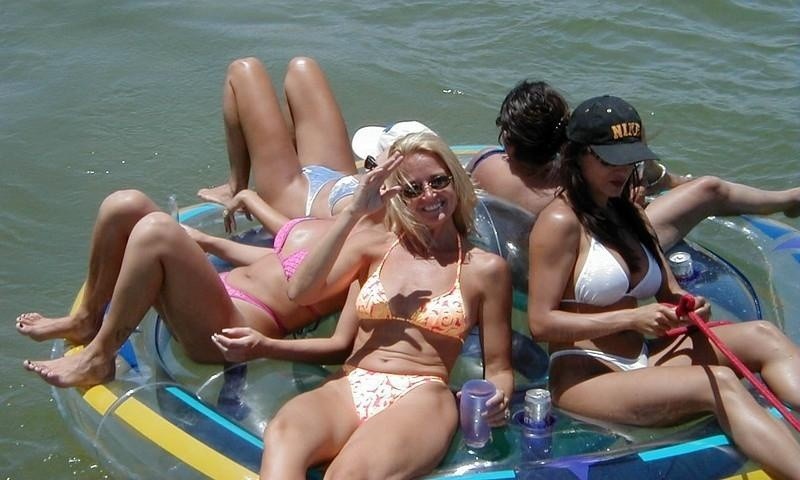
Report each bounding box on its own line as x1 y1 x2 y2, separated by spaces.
504 410 511 420
223 210 229 218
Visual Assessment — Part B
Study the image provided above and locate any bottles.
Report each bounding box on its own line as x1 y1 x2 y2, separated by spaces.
460 379 496 449
167 193 180 224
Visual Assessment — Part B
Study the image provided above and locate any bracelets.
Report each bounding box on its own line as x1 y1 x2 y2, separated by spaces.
647 163 668 189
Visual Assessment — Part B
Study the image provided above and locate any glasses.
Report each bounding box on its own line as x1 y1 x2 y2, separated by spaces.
402 175 452 198
364 155 378 169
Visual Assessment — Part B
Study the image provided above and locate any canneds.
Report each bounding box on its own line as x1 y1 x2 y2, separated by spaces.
523 388 553 428
668 251 695 282
521 427 552 461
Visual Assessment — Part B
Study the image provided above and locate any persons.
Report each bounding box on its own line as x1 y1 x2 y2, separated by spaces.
526 94 800 480
15 145 397 387
463 79 800 254
259 131 514 480
196 56 440 238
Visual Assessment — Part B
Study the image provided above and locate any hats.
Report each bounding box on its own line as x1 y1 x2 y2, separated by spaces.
566 95 661 166
351 121 438 160
